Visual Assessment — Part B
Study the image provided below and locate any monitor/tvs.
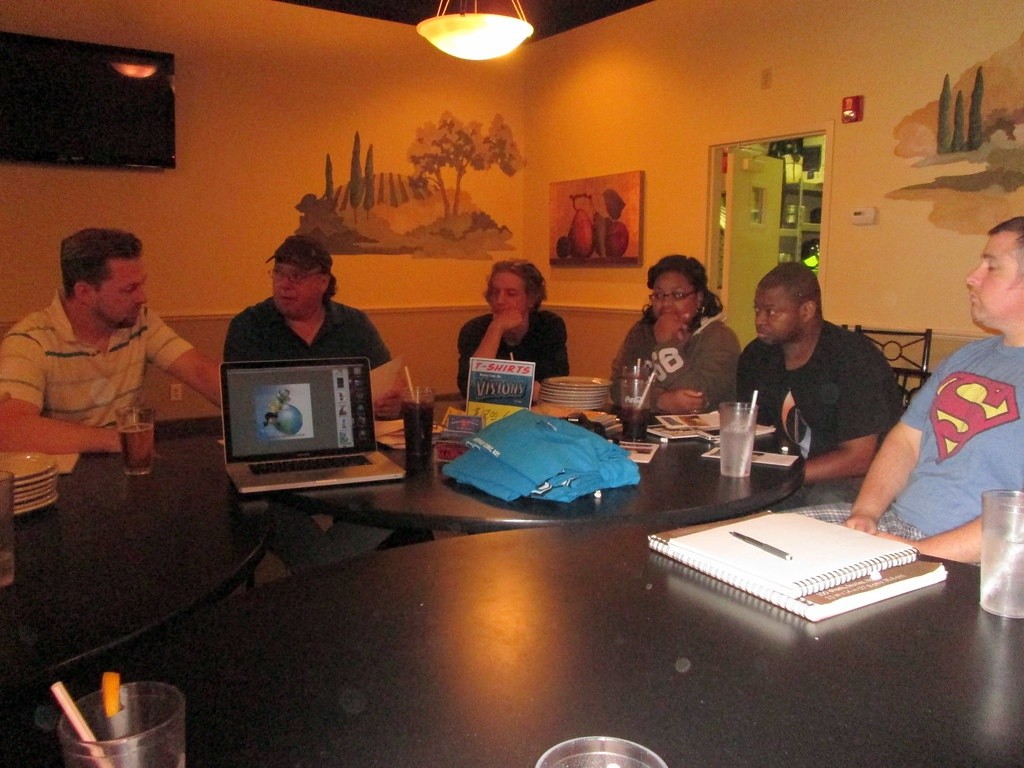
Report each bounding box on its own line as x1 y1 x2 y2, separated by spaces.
0 31 176 170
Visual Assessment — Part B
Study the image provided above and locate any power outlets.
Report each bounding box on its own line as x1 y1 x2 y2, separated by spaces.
170 384 181 401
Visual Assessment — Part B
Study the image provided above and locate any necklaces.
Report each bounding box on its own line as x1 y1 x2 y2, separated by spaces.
735 262 900 511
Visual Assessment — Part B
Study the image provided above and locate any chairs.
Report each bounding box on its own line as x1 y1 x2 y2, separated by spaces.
853 326 933 407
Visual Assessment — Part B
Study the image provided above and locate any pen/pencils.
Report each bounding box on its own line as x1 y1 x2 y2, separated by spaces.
728 531 793 560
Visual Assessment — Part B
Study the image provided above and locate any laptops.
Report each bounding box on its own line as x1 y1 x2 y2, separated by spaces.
219 356 406 492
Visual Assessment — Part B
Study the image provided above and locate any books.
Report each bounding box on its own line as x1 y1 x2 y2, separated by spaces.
646 511 948 622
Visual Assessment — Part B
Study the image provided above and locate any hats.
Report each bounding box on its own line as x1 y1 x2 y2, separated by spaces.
265 234 333 271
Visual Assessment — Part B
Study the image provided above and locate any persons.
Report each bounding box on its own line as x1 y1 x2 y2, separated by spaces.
456 260 569 402
0 228 220 452
791 213 1024 565
610 255 741 415
223 235 407 421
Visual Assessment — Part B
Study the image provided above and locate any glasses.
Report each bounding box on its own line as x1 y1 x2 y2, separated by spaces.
268 270 322 284
649 289 697 304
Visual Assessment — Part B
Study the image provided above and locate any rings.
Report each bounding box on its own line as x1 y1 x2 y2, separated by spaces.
694 410 696 413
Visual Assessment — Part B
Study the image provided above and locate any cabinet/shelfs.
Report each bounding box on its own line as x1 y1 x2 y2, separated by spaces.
779 177 823 262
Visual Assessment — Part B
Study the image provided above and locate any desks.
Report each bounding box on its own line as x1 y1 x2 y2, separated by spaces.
218 392 805 528
0 432 279 686
43 523 1024 768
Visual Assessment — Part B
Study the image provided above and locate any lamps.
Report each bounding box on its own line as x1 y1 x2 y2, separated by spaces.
414 0 536 62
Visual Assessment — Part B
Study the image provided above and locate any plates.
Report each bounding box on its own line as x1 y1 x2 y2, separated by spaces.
0 452 59 514
539 376 612 410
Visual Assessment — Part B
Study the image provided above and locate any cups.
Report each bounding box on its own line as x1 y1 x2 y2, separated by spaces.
535 736 667 767
617 366 653 441
0 470 15 586
57 681 186 768
115 407 156 474
718 402 759 477
403 390 434 454
980 489 1024 619
